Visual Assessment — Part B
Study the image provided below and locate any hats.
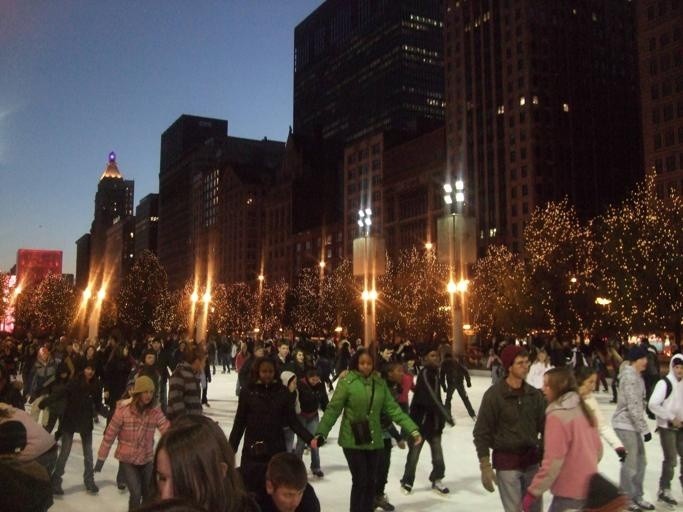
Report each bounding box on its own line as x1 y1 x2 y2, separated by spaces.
0 421 26 455
134 376 154 394
629 346 645 361
580 473 627 512
500 345 525 369
280 371 295 387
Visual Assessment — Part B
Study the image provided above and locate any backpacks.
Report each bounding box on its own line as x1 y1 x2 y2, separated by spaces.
645 377 671 420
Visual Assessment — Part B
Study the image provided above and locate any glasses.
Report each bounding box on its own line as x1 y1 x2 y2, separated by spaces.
519 361 530 368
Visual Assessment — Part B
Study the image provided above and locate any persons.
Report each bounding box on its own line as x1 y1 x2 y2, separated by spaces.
1 336 683 512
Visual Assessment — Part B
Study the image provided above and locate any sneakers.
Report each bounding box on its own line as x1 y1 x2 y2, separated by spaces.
86 485 97 493
400 482 411 492
54 490 63 495
117 479 125 489
372 495 393 512
627 503 641 512
431 480 448 494
656 489 676 505
312 471 323 477
634 501 654 510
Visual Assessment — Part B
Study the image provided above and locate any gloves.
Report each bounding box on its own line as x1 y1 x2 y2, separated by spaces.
480 463 498 492
522 493 535 512
93 459 103 473
307 436 323 447
644 433 650 442
614 447 627 462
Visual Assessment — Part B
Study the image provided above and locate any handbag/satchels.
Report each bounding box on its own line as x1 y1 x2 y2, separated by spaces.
350 421 372 446
